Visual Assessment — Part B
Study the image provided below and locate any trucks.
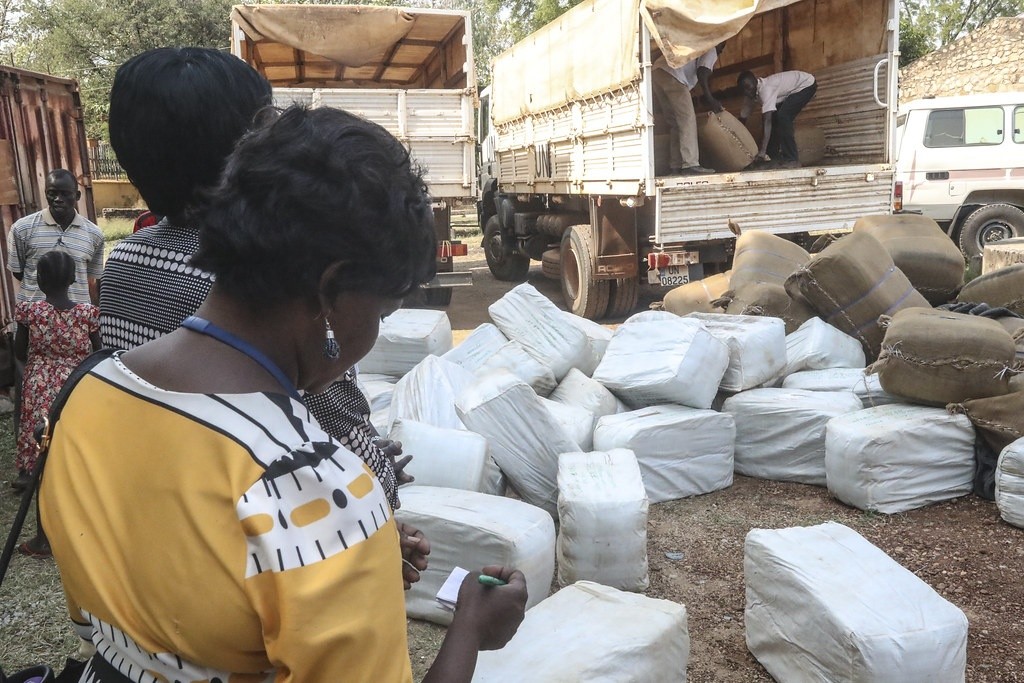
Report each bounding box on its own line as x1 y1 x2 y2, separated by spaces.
472 0 902 325
892 89 1023 269
228 3 479 296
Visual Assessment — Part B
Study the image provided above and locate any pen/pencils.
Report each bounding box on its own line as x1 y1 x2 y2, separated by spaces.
478 574 507 586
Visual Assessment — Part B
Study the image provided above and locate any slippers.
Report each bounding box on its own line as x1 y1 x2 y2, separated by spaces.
16 540 54 560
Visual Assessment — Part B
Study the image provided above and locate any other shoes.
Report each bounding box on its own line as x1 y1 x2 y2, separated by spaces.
671 166 681 175
680 164 716 177
10 471 31 489
781 159 803 170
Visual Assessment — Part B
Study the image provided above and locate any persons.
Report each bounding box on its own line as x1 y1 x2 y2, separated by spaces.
14 250 100 559
6 168 105 489
737 69 817 170
98 47 414 517
35 103 529 683
652 40 728 174
132 210 158 235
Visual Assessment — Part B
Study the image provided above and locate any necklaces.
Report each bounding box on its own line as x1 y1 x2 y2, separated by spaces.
179 315 307 407
